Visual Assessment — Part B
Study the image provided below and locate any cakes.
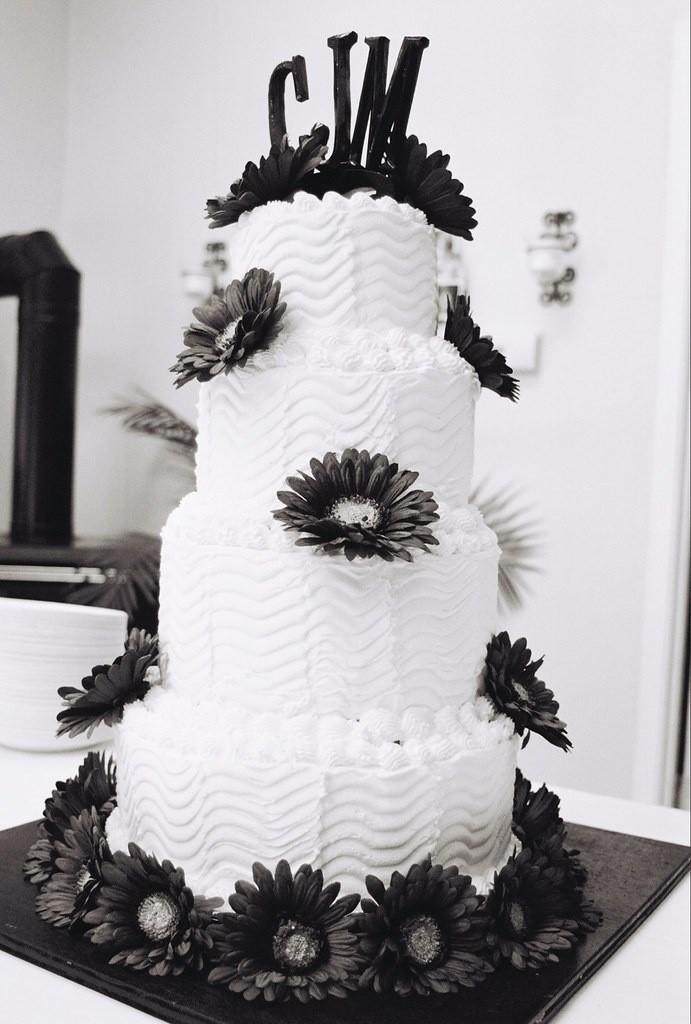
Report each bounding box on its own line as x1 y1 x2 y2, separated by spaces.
102 191 521 917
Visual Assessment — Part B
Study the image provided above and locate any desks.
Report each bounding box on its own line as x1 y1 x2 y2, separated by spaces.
0 723 691 1024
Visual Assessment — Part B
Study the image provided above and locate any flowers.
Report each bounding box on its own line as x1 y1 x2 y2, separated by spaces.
204 860 371 1005
347 852 495 997
82 843 225 977
521 822 602 933
32 804 114 929
204 124 332 228
443 293 521 403
512 767 590 877
169 267 288 390
53 629 160 739
271 448 441 564
365 131 479 242
483 630 574 754
43 748 116 814
484 842 587 970
22 775 88 883
